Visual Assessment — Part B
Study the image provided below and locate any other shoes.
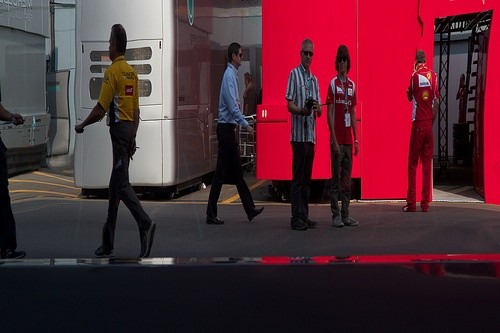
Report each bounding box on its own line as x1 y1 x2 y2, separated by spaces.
301 218 315 227
137 221 156 259
420 203 430 211
402 204 416 212
95 244 113 257
0 249 25 259
290 218 308 230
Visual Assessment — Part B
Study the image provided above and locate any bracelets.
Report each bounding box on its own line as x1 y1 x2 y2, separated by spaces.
9 114 13 122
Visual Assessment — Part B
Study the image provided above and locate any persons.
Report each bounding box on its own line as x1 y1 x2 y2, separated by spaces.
74 24 157 258
242 72 258 120
0 103 26 258
402 50 439 212
285 39 322 229
325 45 359 227
206 42 264 225
456 73 466 125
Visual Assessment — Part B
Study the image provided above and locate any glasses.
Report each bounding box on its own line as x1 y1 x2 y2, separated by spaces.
236 53 242 58
302 50 313 57
338 57 347 63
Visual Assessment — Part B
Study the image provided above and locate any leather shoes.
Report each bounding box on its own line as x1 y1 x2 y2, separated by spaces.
205 217 225 224
248 207 264 220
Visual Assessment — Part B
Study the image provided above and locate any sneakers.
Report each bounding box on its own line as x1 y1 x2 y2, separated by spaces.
333 216 344 227
341 217 360 225
